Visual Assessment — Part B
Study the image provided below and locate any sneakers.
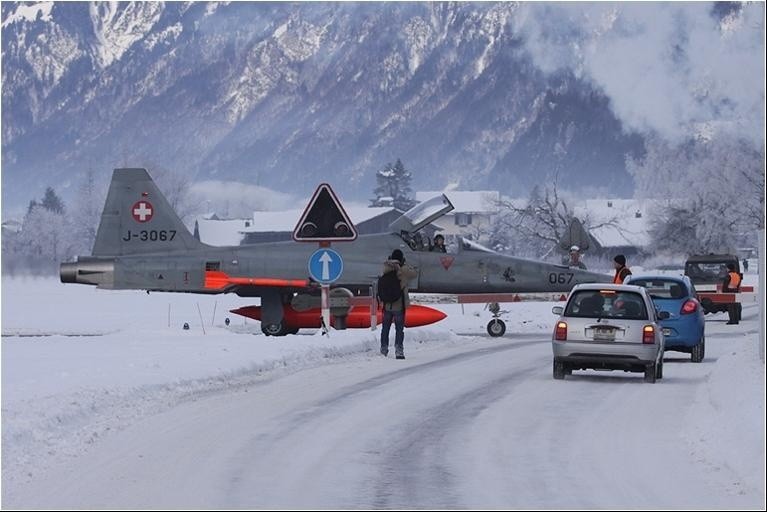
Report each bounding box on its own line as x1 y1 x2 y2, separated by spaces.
725 321 739 325
380 352 405 360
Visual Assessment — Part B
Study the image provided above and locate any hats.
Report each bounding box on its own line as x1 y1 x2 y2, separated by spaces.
377 269 402 303
391 249 402 260
613 254 625 266
726 263 733 269
434 233 444 245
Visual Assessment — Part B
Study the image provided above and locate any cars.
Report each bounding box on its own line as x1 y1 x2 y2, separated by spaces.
551 253 743 383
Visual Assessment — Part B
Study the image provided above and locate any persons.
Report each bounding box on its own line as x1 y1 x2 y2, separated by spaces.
742 258 748 273
722 263 742 325
610 254 633 310
379 249 418 360
431 234 448 255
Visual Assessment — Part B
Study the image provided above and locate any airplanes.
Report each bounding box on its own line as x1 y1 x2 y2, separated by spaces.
60 168 613 337
